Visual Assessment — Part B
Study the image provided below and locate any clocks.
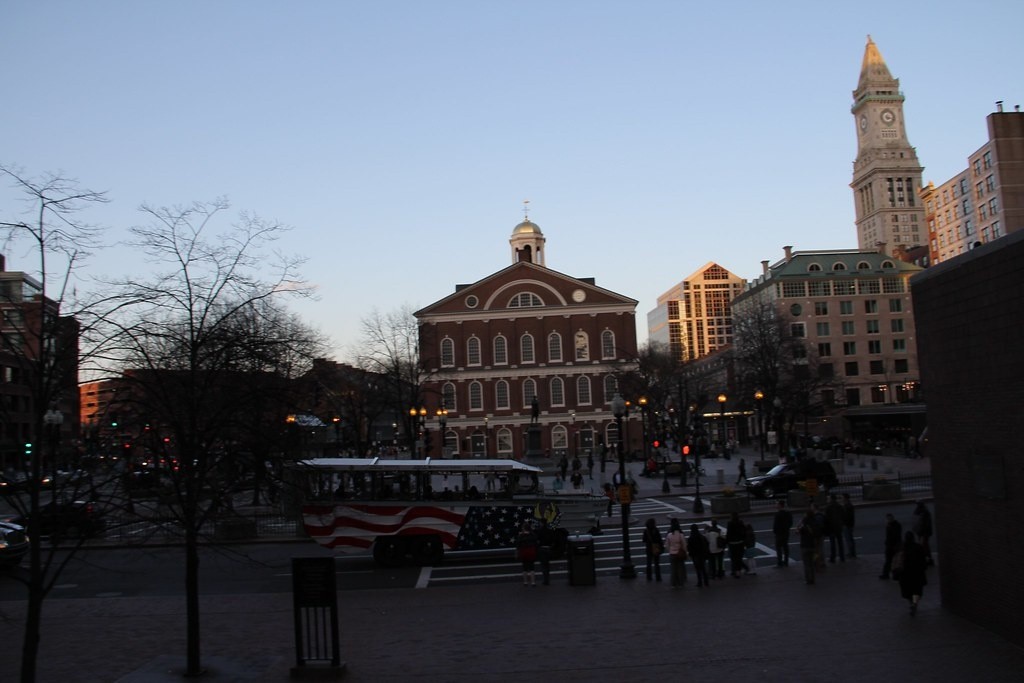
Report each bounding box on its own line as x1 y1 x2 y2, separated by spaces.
859 116 868 130
880 109 896 126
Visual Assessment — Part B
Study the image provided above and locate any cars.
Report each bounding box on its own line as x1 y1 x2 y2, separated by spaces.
0 521 30 571
4 500 107 539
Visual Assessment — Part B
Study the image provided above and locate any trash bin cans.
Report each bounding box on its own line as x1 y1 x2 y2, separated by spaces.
566 531 596 585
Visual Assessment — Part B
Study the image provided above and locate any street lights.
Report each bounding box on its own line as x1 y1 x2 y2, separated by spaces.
754 390 764 461
625 401 631 454
718 394 731 459
409 407 427 460
610 388 638 579
437 409 449 447
638 397 648 477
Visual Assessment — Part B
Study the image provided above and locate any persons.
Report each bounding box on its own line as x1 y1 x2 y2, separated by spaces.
878 513 902 580
665 512 756 586
550 452 594 490
767 431 916 466
794 493 857 586
642 519 664 583
647 456 659 476
913 501 933 558
604 483 616 518
425 485 483 501
734 458 747 485
609 444 617 458
772 501 793 567
531 396 541 423
338 443 401 458
514 517 556 587
892 530 928 613
651 421 736 461
511 477 524 492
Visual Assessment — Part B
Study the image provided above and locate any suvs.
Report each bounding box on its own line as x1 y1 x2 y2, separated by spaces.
743 460 838 500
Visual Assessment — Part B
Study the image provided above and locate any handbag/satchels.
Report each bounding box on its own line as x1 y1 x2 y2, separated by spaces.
677 548 687 562
890 551 905 572
716 536 726 548
